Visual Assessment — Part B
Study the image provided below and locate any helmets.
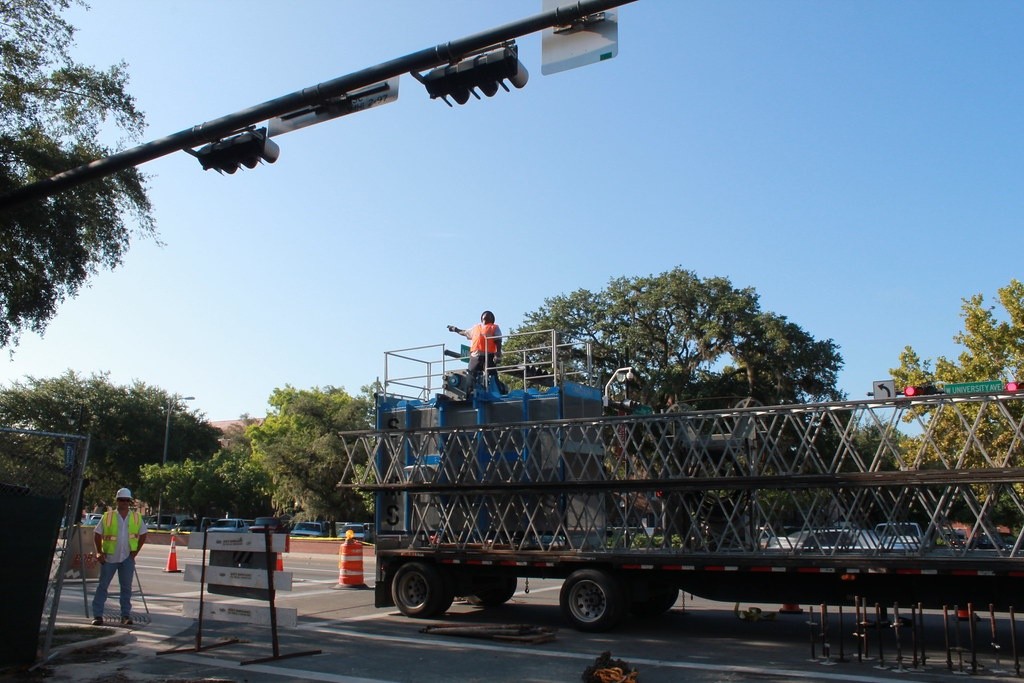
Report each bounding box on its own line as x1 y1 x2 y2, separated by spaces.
116 488 132 498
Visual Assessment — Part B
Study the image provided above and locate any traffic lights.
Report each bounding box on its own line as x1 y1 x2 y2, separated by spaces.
1006 381 1024 392
903 386 936 396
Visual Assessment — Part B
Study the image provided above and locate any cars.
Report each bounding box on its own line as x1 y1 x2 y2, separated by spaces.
206 519 249 533
977 531 1019 550
875 523 930 549
175 517 211 532
291 522 329 537
767 527 874 549
89 515 104 525
341 523 366 541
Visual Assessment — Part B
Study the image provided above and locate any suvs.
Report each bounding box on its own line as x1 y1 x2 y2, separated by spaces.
146 514 177 530
248 516 285 535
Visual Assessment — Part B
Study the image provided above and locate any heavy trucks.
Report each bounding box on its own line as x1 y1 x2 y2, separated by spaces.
368 543 1023 631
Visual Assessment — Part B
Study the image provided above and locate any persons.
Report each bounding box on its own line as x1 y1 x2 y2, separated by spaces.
446 310 508 402
91 487 148 626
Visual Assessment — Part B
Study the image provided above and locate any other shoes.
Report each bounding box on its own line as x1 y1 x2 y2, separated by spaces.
91 617 103 624
119 617 132 624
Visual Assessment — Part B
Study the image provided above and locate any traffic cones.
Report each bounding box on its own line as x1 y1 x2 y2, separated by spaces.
270 533 285 571
162 535 182 573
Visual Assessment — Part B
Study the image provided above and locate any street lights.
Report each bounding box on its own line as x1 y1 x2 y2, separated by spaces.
164 396 196 466
